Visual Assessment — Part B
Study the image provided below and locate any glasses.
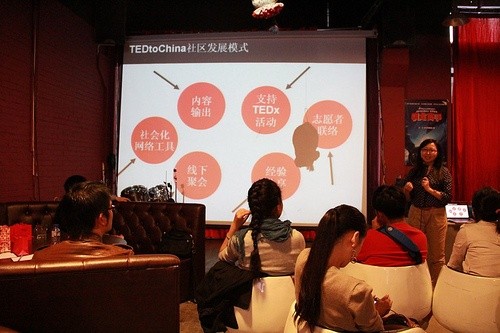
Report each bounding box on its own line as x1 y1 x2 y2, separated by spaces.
107 204 115 211
420 148 437 152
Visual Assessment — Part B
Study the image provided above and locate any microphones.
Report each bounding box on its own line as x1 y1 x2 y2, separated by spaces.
173 169 177 203
423 164 429 180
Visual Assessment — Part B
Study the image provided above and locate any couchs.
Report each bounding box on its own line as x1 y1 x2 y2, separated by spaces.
0 255 181 333
0 202 205 304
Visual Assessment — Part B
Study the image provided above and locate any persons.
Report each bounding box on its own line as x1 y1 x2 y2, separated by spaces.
218 177 305 276
294 203 394 333
401 138 453 287
31 179 137 259
63 173 131 202
446 183 500 278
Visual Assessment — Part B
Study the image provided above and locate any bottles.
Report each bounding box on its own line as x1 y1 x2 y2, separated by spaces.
51 224 60 244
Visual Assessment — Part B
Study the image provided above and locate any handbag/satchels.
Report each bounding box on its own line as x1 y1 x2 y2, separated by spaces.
381 309 422 331
158 228 195 259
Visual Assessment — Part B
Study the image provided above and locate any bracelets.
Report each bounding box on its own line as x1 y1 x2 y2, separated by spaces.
431 189 437 195
226 234 232 240
352 183 428 268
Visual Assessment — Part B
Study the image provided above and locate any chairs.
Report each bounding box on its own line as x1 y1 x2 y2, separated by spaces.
225 262 500 333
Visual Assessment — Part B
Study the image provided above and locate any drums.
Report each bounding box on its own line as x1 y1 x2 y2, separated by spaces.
146 184 171 202
120 183 148 202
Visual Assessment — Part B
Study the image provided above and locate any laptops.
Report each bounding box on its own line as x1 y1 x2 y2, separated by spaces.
445 203 475 223
